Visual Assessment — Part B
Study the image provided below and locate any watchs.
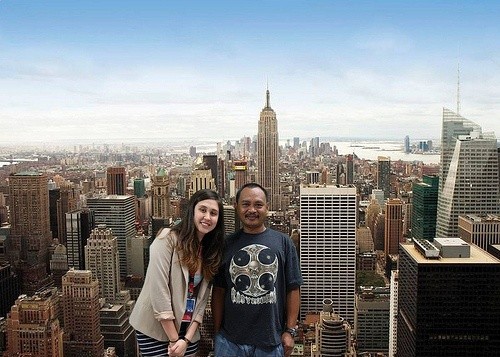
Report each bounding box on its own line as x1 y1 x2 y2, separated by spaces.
285 326 297 337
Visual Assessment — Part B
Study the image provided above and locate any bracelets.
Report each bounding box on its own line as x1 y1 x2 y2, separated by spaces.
180 335 192 346
168 337 181 342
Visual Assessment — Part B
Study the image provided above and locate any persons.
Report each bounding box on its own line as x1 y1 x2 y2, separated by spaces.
209 183 305 357
130 189 225 357
